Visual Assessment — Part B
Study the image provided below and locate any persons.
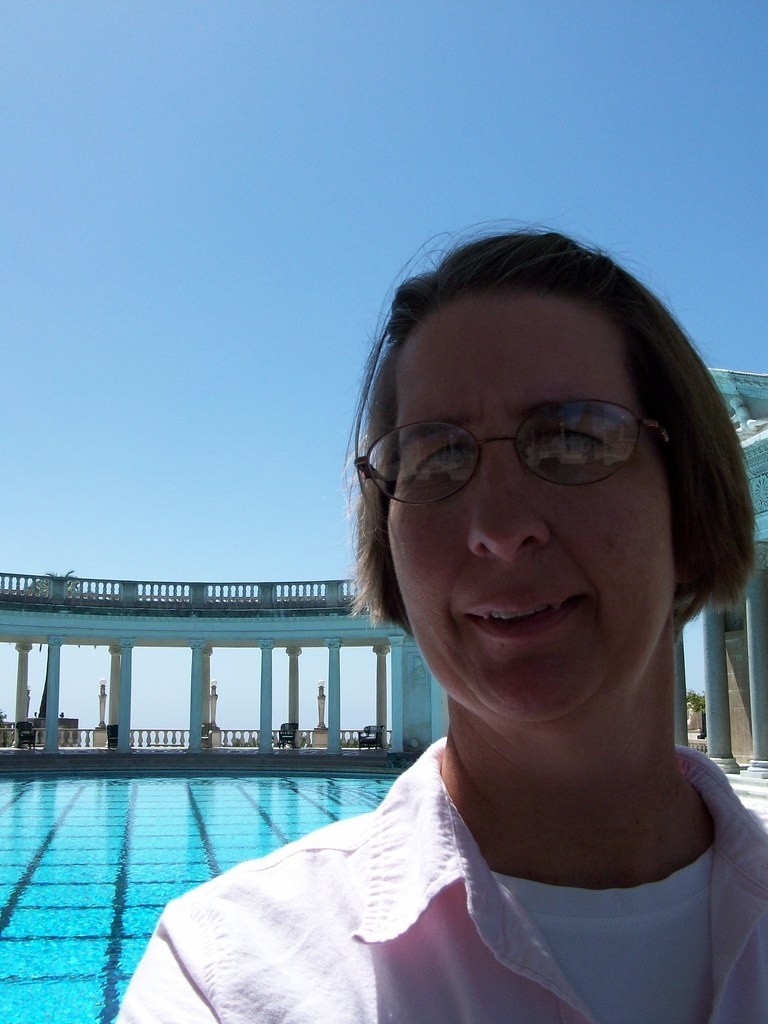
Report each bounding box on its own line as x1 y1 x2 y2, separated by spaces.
110 218 768 1024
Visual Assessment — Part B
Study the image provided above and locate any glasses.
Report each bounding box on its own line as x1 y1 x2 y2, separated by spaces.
351 396 670 506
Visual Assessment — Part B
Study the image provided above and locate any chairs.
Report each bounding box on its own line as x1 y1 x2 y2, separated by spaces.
358 726 386 750
279 722 298 748
15 721 37 750
106 725 118 748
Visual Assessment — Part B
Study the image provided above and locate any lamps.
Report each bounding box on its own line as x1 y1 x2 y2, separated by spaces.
211 680 216 696
317 680 325 697
100 679 107 695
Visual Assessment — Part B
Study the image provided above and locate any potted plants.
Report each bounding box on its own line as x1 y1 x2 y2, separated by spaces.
684 690 706 737
